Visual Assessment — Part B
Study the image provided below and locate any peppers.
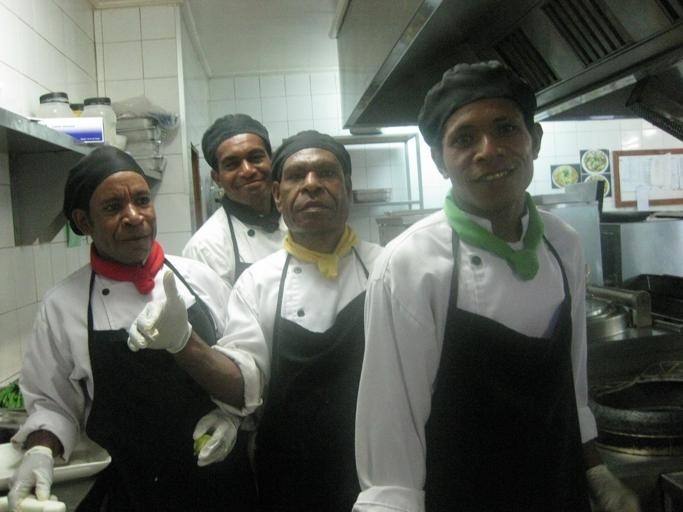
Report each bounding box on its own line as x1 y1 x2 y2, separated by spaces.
192 433 212 457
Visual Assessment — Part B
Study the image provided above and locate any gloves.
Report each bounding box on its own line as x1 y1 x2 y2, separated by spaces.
127 270 193 353
585 463 640 512
192 404 242 467
6 446 66 512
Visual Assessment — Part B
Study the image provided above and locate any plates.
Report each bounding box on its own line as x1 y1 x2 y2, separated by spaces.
0 432 113 493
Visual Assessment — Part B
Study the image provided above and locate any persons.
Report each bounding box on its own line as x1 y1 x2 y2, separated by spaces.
0 140 262 511
177 113 291 292
350 59 646 511
123 129 385 512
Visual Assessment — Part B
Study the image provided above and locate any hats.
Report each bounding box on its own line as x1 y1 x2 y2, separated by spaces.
202 113 271 167
62 143 152 236
417 59 536 147
271 129 352 185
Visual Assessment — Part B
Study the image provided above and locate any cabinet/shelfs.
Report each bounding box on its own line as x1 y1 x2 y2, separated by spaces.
328 132 424 212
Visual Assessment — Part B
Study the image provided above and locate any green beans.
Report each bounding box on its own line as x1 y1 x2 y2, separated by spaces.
0 379 25 410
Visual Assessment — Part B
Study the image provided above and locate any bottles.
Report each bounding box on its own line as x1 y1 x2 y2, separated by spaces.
38 91 117 150
